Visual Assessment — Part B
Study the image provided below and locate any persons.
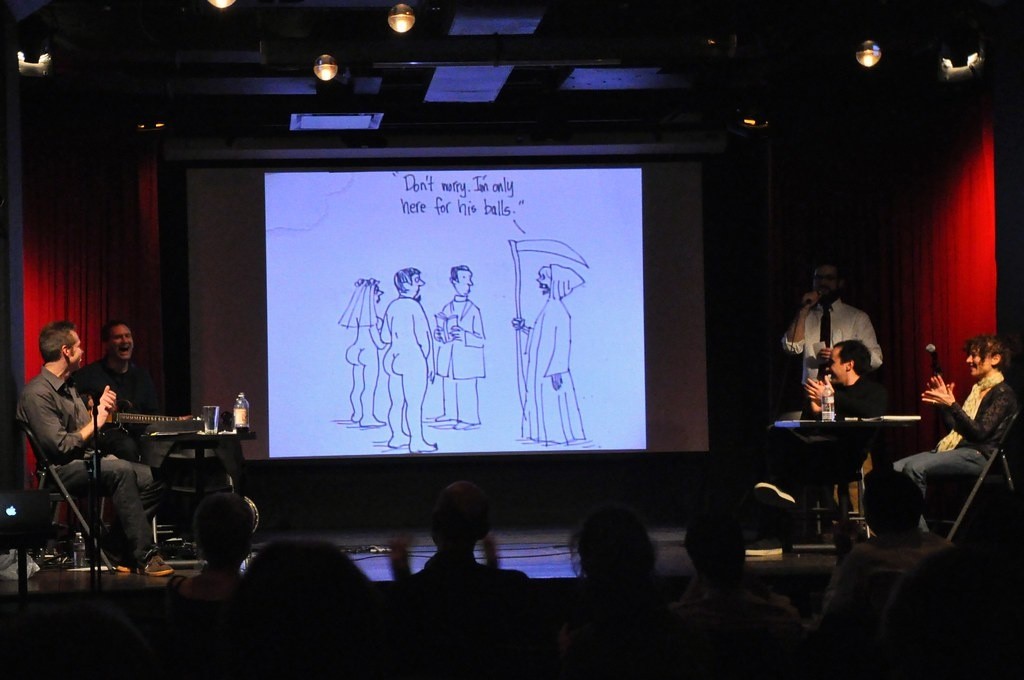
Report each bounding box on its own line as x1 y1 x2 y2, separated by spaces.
64 321 158 415
0 482 1024 680
16 321 175 574
891 335 1016 534
782 262 883 385
785 340 886 543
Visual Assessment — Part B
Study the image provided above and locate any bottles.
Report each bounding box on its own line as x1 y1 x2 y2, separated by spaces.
233 392 250 432
821 385 835 421
73 532 85 568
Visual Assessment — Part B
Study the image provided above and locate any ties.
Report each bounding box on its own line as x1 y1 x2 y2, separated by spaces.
817 305 832 379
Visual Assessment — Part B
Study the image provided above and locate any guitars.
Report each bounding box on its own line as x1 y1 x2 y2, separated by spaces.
86 399 193 440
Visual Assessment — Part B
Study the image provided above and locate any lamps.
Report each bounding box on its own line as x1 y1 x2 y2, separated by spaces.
312 55 337 81
387 4 416 32
16 21 51 70
939 39 977 72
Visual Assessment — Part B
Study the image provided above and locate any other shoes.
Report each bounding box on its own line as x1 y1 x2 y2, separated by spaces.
744 539 785 557
753 481 800 510
99 547 132 575
135 554 174 576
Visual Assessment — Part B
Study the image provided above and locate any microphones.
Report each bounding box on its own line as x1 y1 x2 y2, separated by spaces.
800 290 823 308
926 343 938 369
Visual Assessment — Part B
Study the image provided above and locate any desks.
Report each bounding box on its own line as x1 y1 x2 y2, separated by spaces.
770 413 922 552
150 428 258 569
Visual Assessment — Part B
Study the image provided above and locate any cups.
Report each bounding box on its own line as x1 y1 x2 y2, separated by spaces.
204 405 219 435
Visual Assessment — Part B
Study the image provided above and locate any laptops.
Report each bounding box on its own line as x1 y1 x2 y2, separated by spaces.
0 489 50 534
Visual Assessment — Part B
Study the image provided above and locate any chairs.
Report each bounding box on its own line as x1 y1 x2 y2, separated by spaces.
932 409 1019 541
16 417 122 588
